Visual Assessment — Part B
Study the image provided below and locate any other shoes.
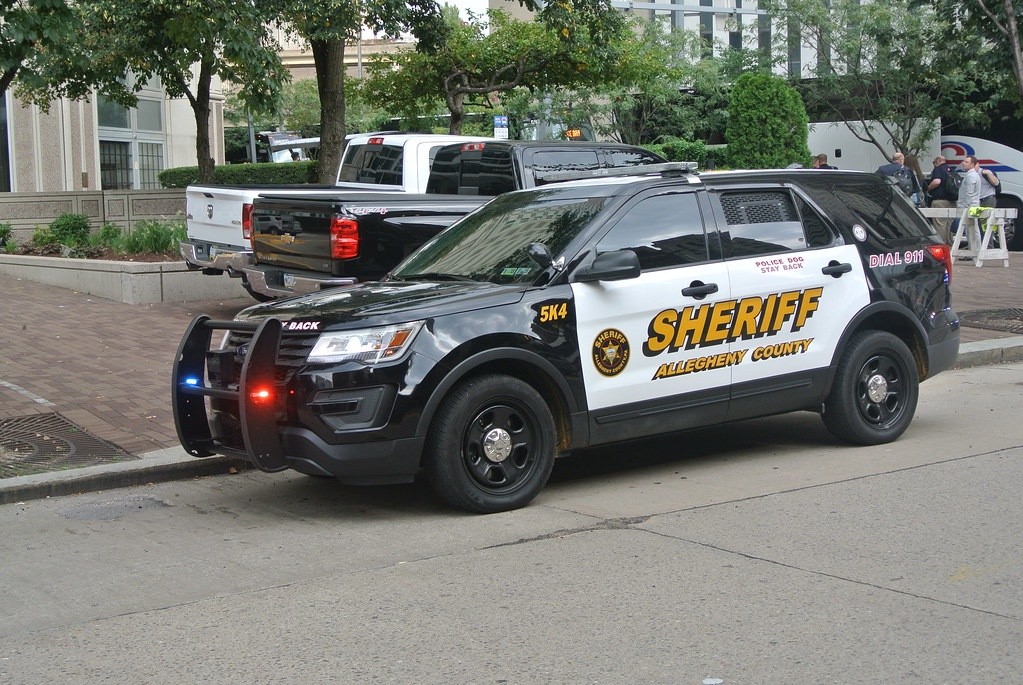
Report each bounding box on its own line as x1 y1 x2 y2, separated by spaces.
958 256 972 261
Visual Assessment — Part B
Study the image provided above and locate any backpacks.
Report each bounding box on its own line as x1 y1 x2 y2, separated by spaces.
981 168 1001 198
892 165 926 209
940 166 962 202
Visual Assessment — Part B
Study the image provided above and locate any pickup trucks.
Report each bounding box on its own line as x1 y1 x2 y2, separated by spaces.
241 140 676 304
179 134 514 281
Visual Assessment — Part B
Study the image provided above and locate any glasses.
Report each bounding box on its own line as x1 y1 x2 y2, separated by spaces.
902 157 905 159
933 161 936 165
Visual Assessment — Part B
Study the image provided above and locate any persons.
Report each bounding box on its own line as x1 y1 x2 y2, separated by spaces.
956 155 1000 261
812 154 838 170
928 155 956 247
876 152 922 196
291 152 301 161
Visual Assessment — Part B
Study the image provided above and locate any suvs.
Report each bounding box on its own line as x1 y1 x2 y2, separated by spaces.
168 162 957 516
